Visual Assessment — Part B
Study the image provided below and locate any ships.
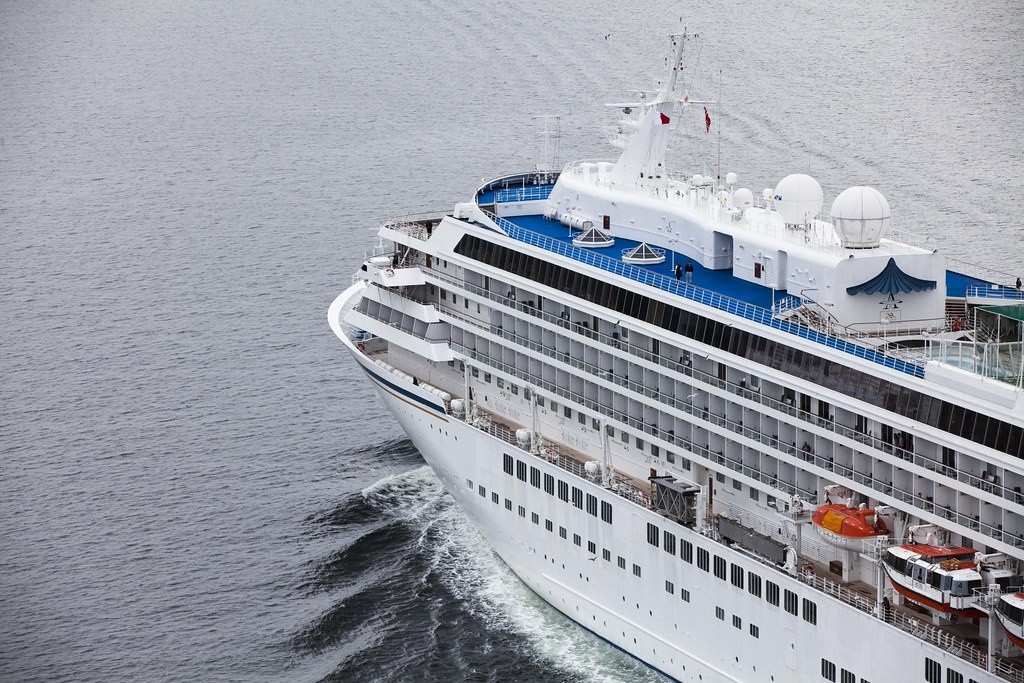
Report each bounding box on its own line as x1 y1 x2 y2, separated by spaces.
327 24 1024 683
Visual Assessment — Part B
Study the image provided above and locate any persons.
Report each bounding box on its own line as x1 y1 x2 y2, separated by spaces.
675 260 694 287
918 492 928 510
801 441 812 461
1016 278 1022 298
894 433 904 459
882 597 891 622
425 220 433 239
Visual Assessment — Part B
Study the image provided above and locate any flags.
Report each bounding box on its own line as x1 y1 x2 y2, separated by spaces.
654 110 671 126
703 106 711 133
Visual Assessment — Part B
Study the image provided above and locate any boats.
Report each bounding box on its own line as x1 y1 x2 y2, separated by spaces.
811 503 890 553
881 544 988 618
994 591 1024 650
